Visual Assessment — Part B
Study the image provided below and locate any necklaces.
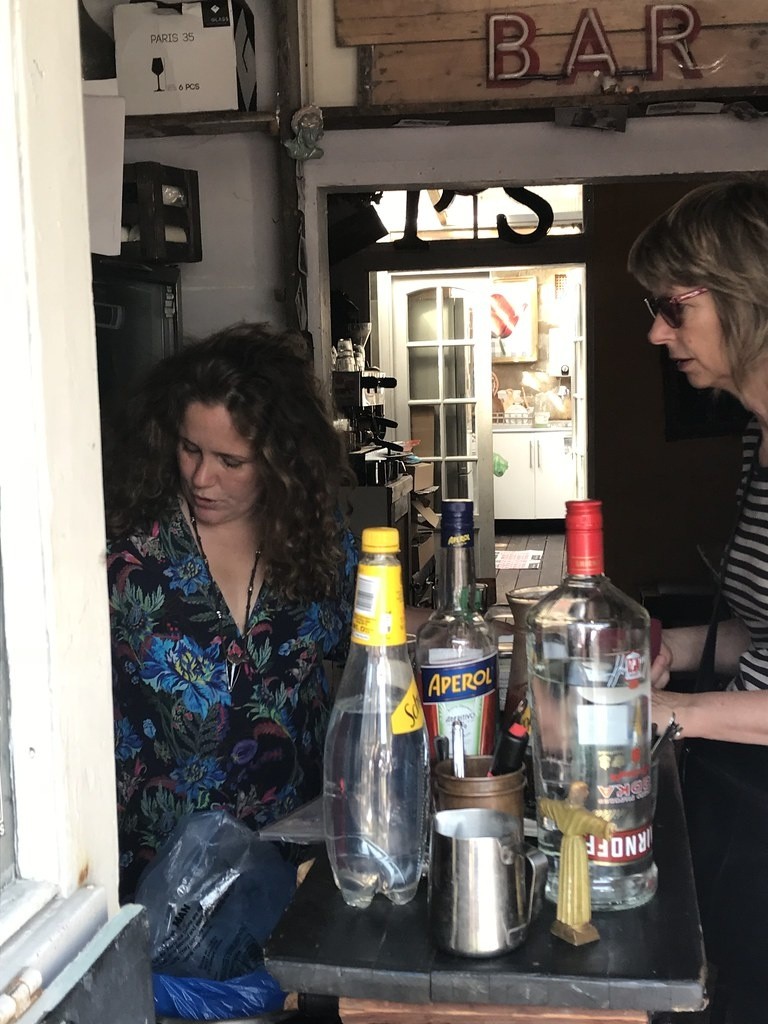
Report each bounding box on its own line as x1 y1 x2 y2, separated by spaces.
188 501 262 657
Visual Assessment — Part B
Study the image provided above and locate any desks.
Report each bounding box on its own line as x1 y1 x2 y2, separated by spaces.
339 473 413 606
260 652 709 1024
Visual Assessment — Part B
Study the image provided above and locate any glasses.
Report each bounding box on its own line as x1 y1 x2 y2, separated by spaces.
644 286 710 329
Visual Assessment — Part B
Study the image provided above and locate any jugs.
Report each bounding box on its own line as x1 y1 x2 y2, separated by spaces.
506 585 560 807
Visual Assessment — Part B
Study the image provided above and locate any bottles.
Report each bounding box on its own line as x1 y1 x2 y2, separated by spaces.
413 497 498 767
526 501 657 912
321 526 432 908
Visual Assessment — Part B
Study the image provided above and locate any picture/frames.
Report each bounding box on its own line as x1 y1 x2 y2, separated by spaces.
660 344 754 443
491 275 539 366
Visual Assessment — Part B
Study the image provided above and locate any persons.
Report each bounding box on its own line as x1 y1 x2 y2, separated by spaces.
106 321 515 886
627 178 768 1023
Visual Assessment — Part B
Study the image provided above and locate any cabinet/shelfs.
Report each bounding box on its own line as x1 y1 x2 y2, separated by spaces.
492 431 577 520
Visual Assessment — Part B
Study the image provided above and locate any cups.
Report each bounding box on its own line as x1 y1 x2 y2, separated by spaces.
428 808 549 959
433 756 525 826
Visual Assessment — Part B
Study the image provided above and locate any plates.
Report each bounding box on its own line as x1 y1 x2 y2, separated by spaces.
504 406 528 424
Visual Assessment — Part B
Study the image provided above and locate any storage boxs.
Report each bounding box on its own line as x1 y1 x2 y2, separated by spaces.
400 405 443 606
119 161 202 262
113 0 257 115
